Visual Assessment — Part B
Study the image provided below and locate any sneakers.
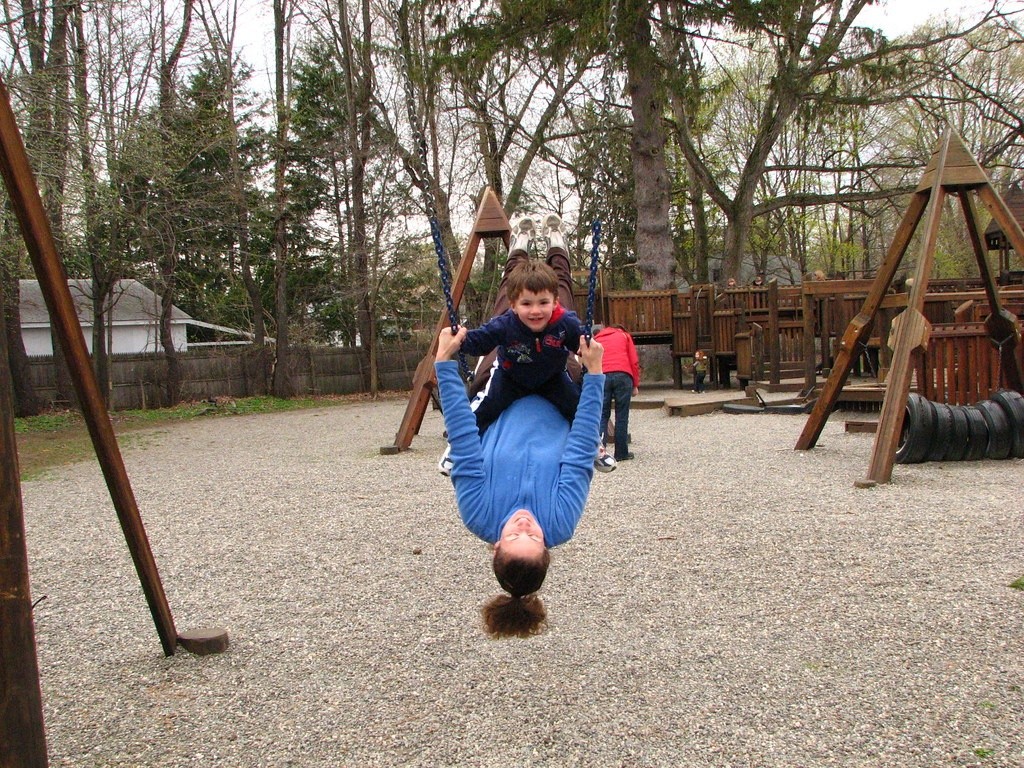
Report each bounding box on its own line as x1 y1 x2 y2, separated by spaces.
508 216 538 262
541 212 570 265
438 442 453 477
613 451 635 461
593 432 617 474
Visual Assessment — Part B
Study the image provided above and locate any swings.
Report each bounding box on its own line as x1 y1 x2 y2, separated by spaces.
387 0 621 403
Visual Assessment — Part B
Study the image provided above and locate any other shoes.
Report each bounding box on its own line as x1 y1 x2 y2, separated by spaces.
699 391 705 394
692 390 697 395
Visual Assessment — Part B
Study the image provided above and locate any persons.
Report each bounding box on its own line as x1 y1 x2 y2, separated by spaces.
591 324 640 462
434 213 606 639
438 258 616 477
691 350 707 393
